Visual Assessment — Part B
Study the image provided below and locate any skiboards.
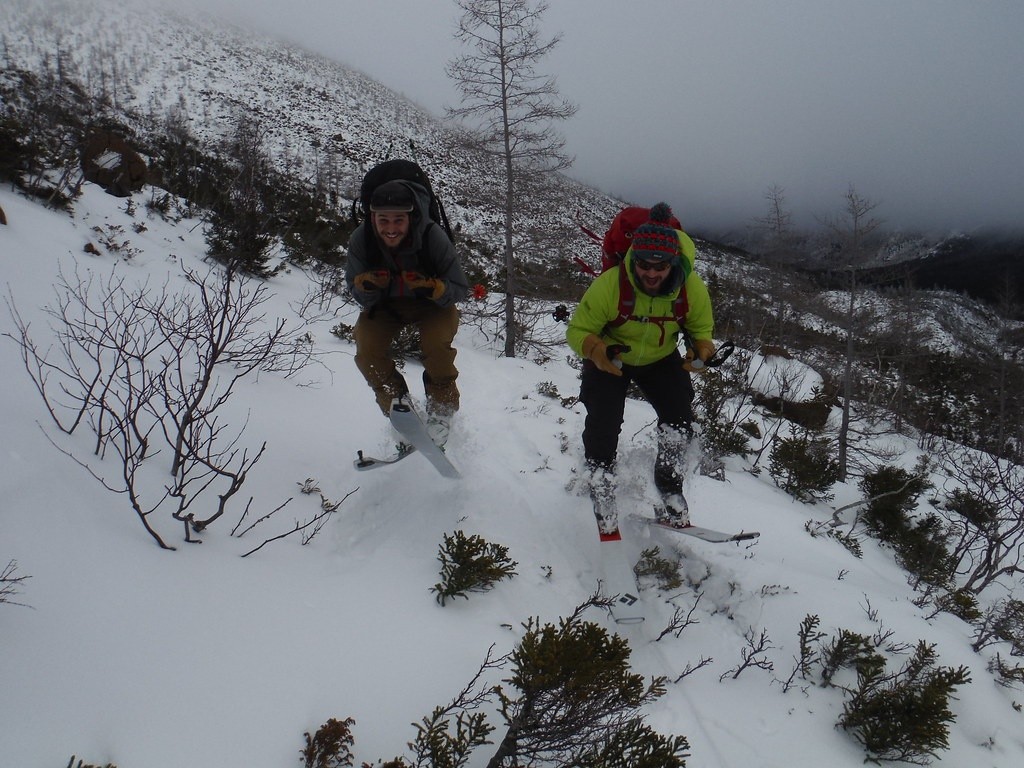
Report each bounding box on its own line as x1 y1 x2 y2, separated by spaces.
353 397 462 481
593 486 760 627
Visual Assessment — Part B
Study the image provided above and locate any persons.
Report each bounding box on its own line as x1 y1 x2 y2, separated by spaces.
565 202 715 535
345 180 469 449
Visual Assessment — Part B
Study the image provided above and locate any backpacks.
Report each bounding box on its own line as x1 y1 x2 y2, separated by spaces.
601 206 689 335
359 158 440 270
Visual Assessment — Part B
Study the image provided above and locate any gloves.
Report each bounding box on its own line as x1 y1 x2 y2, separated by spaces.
353 270 398 293
392 272 446 300
682 340 714 373
582 334 622 376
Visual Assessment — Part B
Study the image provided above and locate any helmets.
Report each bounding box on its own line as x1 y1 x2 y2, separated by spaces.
370 183 416 213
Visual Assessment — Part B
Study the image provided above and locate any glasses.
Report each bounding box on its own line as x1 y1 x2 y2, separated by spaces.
633 254 671 272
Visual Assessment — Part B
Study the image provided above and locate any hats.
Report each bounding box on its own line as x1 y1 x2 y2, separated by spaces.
632 202 683 260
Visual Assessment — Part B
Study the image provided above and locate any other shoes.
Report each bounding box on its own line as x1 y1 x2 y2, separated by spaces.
656 486 688 518
426 400 455 447
587 459 618 520
391 387 414 413
